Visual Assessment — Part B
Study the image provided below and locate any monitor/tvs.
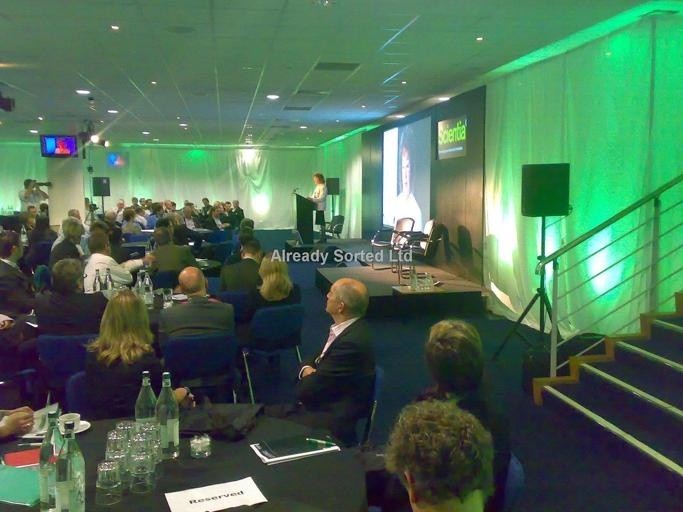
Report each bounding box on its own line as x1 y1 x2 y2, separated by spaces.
40 134 78 159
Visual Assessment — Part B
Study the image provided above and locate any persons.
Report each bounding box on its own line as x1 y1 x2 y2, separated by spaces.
223 226 253 267
261 276 378 447
0 406 34 439
218 203 227 217
146 199 152 205
157 265 237 356
150 225 209 289
187 203 203 219
83 288 165 419
48 216 86 273
153 218 173 250
233 198 244 219
232 218 260 247
412 318 492 427
166 213 200 251
19 179 49 214
224 201 239 224
385 400 495 511
145 203 163 228
237 252 302 325
83 227 155 294
1 228 34 311
140 198 148 209
121 209 141 234
85 221 109 254
34 258 108 337
25 206 40 237
58 209 89 235
307 172 329 244
220 239 266 292
182 206 199 230
0 311 15 332
203 206 234 229
200 198 213 216
112 200 125 226
105 212 121 245
131 198 139 209
31 215 57 244
391 125 424 233
134 206 146 226
164 200 173 213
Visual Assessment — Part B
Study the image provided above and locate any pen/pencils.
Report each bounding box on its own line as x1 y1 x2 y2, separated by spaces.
18 441 43 447
306 438 336 446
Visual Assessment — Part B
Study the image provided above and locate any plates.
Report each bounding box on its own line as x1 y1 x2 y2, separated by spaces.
13 420 91 439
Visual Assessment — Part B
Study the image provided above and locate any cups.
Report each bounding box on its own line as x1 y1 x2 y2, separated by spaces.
140 424 163 464
131 433 156 474
95 462 121 505
130 455 152 494
417 272 441 290
164 288 174 303
190 437 211 458
58 413 80 433
105 430 130 474
116 421 136 442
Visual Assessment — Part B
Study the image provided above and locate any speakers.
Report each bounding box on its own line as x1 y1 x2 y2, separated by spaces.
521 163 570 219
327 178 339 195
92 177 112 197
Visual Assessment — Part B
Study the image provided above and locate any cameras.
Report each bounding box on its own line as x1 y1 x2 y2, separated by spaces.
33 180 51 187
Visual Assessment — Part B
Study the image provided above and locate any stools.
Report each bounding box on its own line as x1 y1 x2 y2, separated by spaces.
0 369 37 410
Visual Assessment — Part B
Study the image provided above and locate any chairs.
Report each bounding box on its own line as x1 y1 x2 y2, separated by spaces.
398 226 441 286
198 243 234 263
34 264 50 292
237 305 310 405
337 367 384 451
152 270 180 290
23 242 51 274
37 336 98 415
216 291 254 307
65 372 93 422
204 255 240 296
391 218 437 277
320 215 344 239
371 217 415 273
157 334 237 404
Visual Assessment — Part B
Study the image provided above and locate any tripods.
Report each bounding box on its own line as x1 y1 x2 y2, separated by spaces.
488 217 567 367
326 195 341 240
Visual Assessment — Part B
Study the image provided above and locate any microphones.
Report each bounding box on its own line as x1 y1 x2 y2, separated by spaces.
291 188 298 193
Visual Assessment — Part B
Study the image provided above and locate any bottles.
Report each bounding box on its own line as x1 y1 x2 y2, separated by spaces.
39 411 63 512
93 269 103 291
134 271 144 305
144 270 153 304
154 372 179 458
134 371 157 421
104 268 114 290
56 420 85 512
